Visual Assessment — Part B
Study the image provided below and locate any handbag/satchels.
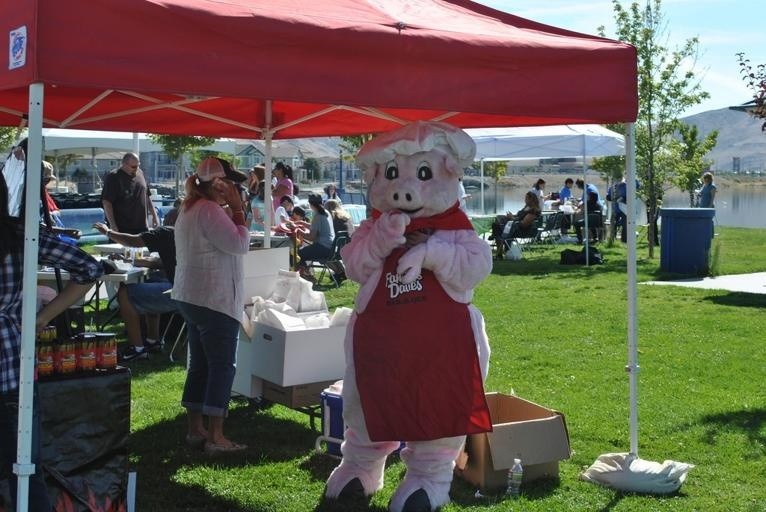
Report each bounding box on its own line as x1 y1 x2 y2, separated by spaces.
560 246 603 265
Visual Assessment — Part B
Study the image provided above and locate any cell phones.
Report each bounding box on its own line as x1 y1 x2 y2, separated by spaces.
212 178 228 187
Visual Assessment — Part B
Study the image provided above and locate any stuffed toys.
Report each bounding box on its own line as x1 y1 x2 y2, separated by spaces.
318 117 495 511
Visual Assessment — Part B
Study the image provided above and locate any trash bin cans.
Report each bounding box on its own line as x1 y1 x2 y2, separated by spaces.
660 209 715 275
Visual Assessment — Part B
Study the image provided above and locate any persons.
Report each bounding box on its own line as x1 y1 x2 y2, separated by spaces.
41 160 82 240
235 159 354 274
484 171 665 257
163 156 255 454
88 206 181 363
0 172 105 508
163 200 182 226
699 172 718 208
99 152 160 235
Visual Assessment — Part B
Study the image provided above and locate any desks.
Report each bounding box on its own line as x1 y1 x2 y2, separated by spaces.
33 258 153 363
94 235 161 258
0 365 134 512
246 229 312 253
341 204 366 227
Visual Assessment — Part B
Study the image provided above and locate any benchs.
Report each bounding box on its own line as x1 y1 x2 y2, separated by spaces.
52 208 107 247
154 205 174 226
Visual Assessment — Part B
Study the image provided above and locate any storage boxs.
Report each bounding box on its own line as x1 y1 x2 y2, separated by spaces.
248 319 352 389
257 380 337 410
184 336 237 400
230 332 263 399
455 386 573 496
319 377 407 461
232 246 293 322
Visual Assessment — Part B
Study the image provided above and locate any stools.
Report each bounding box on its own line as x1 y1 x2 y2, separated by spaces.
36 302 86 351
160 308 191 362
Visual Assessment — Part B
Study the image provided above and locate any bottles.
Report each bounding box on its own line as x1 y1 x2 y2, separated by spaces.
504 457 524 497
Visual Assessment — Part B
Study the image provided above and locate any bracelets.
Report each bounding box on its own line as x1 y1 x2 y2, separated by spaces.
105 230 112 236
220 204 229 209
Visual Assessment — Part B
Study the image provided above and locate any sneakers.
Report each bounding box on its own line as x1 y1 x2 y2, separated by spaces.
118 344 149 365
146 337 165 351
186 433 209 444
205 441 247 453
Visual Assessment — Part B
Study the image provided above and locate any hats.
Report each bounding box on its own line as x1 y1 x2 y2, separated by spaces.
196 155 247 182
308 193 322 203
100 259 128 274
43 161 57 181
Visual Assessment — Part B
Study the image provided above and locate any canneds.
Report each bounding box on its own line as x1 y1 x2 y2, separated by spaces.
36 325 118 379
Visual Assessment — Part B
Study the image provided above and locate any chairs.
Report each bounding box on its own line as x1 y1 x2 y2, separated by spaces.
299 230 351 290
476 199 663 264
